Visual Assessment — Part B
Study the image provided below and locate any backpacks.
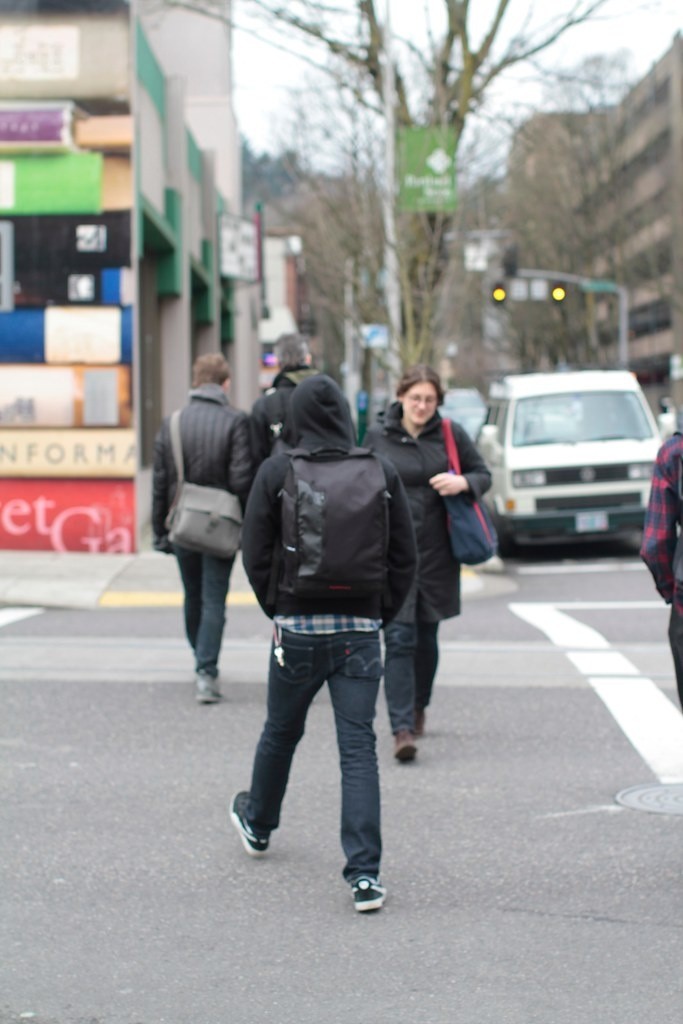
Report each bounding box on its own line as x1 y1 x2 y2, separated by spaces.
279 448 388 598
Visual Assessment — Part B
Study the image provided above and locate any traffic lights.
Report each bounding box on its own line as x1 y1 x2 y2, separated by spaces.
489 274 509 307
548 276 567 304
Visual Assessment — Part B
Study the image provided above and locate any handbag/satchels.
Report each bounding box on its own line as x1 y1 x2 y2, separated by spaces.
439 418 497 566
164 482 244 560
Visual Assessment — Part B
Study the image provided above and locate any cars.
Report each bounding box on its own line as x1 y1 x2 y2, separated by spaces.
437 386 488 442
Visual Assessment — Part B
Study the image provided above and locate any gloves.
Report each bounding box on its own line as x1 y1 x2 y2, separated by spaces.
153 532 175 555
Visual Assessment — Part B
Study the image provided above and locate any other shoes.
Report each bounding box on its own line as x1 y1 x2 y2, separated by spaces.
196 684 223 701
411 709 425 733
395 729 418 762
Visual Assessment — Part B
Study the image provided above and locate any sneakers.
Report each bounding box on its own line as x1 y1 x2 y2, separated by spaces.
229 790 269 856
352 874 386 911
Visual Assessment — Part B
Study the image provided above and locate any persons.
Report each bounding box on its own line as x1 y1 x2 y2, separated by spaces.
358 367 493 762
149 351 252 703
250 331 321 462
230 373 417 911
640 430 682 712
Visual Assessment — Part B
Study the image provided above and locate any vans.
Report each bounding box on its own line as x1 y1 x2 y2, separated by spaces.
473 368 676 562
